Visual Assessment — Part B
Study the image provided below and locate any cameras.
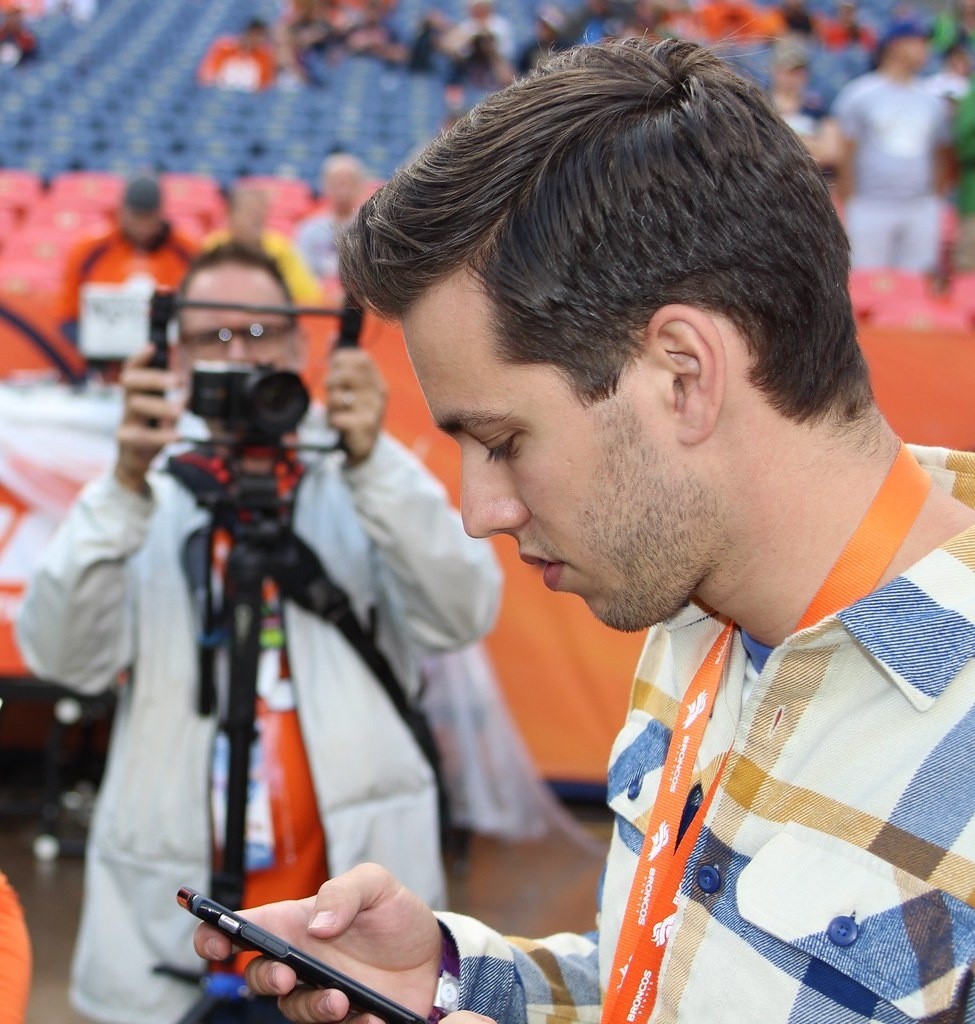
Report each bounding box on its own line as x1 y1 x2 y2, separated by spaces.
186 363 308 434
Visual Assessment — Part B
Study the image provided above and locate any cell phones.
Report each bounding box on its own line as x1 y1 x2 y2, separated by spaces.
175 884 431 1024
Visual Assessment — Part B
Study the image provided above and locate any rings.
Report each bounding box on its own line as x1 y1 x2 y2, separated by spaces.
345 392 351 406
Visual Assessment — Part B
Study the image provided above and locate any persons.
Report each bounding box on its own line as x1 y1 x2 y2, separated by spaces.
19 238 504 1023
194 38 974 1023
296 156 371 278
0 1 97 71
58 179 196 367
202 190 322 302
196 0 975 277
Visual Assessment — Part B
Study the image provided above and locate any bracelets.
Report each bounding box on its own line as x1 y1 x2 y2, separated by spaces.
428 940 458 1023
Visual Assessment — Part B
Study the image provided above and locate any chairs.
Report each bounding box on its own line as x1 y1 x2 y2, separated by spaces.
0 0 974 797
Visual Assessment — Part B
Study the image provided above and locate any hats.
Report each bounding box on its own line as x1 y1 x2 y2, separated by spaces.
880 18 936 42
124 177 159 212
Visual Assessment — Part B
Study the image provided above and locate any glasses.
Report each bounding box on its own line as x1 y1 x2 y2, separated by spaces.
180 321 292 359
